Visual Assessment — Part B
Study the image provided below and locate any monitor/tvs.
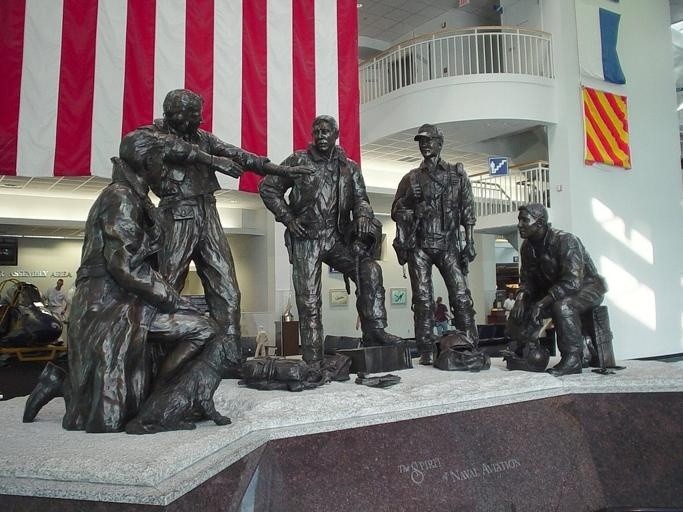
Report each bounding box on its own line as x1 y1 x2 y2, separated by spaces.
0 239 19 266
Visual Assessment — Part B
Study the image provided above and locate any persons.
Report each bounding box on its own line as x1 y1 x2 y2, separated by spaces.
22 129 223 433
391 123 479 365
41 279 69 343
255 115 402 363
503 292 517 320
435 297 448 335
252 326 268 358
137 88 318 348
503 202 606 376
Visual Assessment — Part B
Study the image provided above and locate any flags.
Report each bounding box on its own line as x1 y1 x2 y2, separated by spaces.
575 0 626 85
581 86 631 169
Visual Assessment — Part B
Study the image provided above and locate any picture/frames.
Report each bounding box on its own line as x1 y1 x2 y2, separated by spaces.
328 288 350 307
390 287 408 306
